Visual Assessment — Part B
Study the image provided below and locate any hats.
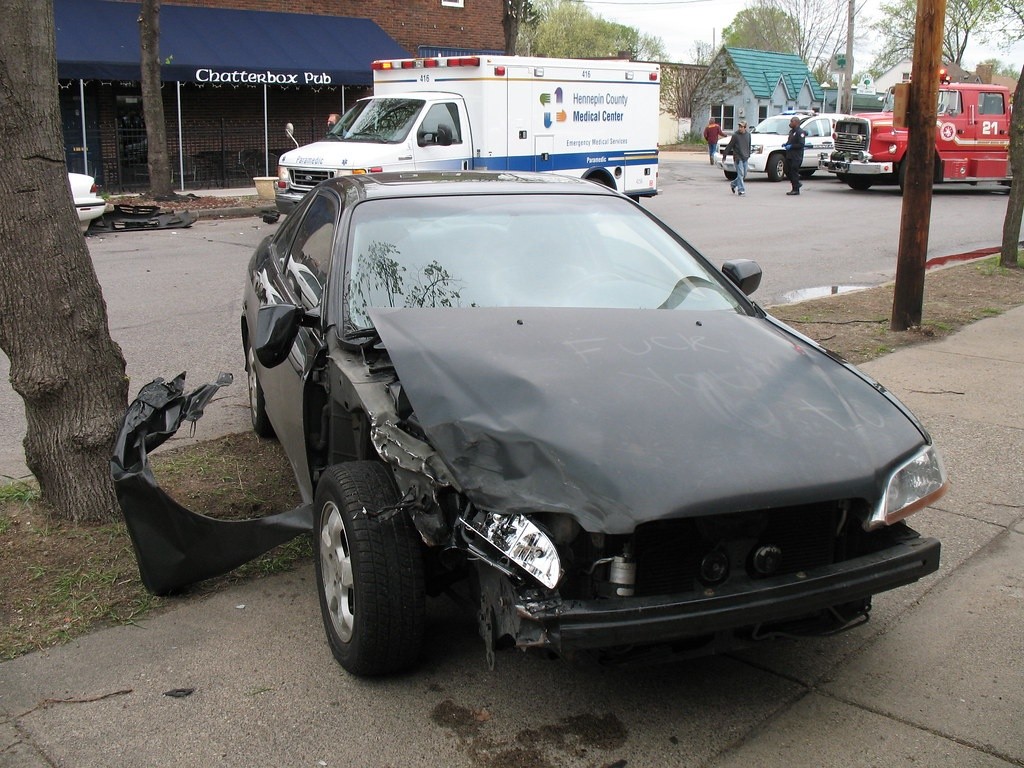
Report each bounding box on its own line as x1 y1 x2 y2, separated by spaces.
738 120 747 126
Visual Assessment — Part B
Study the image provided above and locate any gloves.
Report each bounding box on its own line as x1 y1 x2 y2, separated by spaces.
782 143 787 147
785 145 791 150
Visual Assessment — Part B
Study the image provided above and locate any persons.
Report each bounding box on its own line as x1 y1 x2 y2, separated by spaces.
704 117 727 165
781 117 805 195
722 120 751 196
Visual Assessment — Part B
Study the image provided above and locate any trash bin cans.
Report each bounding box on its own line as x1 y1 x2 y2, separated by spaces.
252 176 280 200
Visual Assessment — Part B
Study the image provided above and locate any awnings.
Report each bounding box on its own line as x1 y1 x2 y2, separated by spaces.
53 1 414 86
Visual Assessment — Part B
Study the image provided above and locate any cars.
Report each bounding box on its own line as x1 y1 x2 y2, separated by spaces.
713 110 852 180
109 166 951 682
67 172 107 235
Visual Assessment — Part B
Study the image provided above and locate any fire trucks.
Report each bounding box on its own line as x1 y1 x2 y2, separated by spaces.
274 47 664 213
819 67 1013 194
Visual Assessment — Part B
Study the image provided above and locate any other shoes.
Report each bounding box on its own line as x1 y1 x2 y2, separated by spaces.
786 190 799 195
710 156 714 165
799 183 802 188
732 188 735 193
739 192 745 196
